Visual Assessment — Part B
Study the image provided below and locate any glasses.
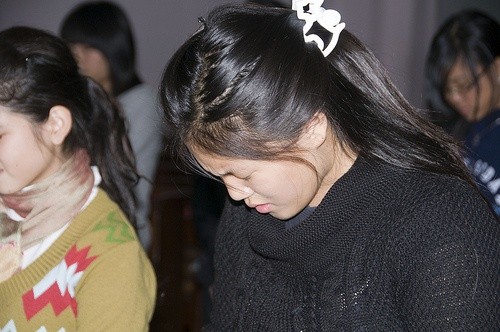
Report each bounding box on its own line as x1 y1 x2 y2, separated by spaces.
443 67 492 92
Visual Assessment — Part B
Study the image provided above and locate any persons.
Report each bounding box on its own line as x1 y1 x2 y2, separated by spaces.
0 26 157 332
160 0 500 332
60 0 168 251
425 8 500 219
